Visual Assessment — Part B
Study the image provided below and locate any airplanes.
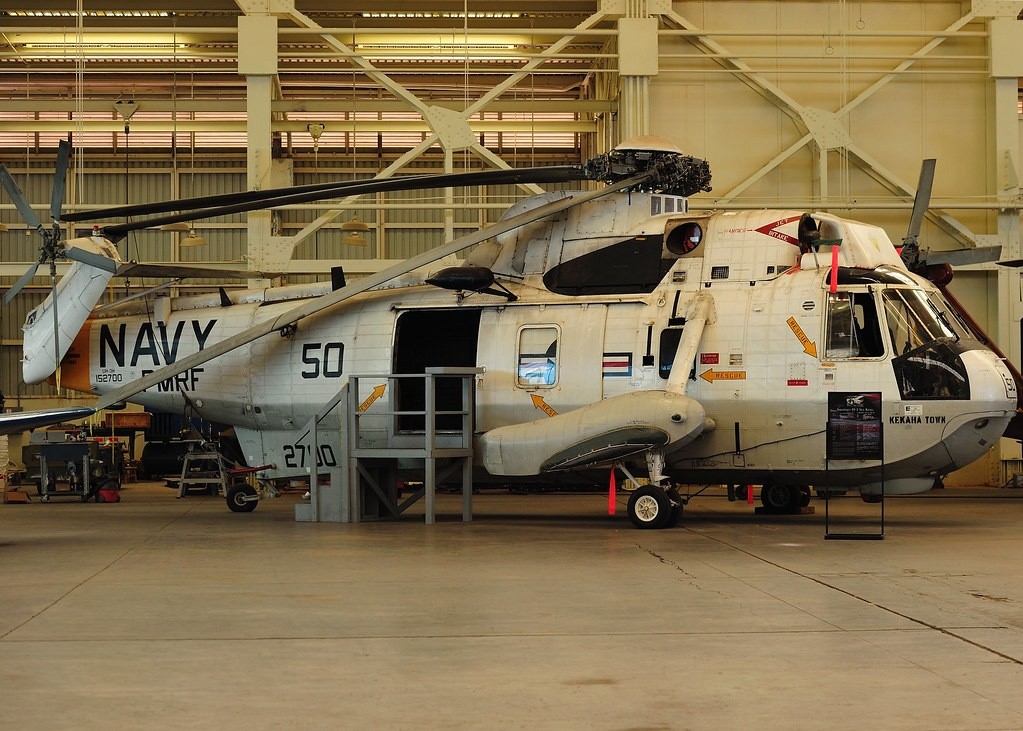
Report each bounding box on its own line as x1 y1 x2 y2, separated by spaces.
2 133 1023 548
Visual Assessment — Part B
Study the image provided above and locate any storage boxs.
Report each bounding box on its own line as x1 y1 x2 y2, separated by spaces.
105 412 152 428
3 490 31 504
54 482 70 492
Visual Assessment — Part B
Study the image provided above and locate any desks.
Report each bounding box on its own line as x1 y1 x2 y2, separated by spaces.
176 453 228 499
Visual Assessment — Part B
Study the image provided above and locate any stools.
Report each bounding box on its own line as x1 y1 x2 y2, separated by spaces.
120 466 138 483
